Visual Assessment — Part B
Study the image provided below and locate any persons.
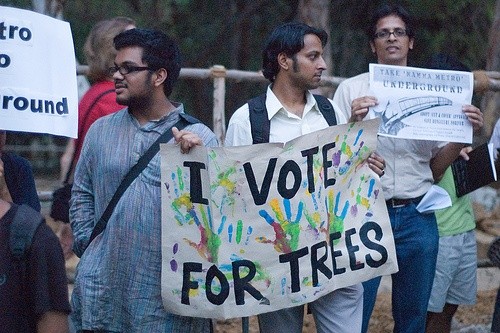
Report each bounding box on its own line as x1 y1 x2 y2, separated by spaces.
482 92 500 333
0 130 41 213
0 199 72 333
224 22 387 333
56 17 137 282
69 27 219 333
425 61 477 333
333 7 484 333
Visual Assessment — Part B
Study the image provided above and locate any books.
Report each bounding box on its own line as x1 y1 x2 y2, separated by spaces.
451 142 497 198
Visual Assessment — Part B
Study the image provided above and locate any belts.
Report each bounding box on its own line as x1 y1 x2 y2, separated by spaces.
386 193 427 208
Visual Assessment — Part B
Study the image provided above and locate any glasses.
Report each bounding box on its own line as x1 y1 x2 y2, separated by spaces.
373 28 408 37
109 65 157 74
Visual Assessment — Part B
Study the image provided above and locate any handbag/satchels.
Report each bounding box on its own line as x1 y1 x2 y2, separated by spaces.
49 183 72 222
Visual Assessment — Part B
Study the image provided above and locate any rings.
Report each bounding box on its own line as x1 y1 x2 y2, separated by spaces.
379 169 385 176
